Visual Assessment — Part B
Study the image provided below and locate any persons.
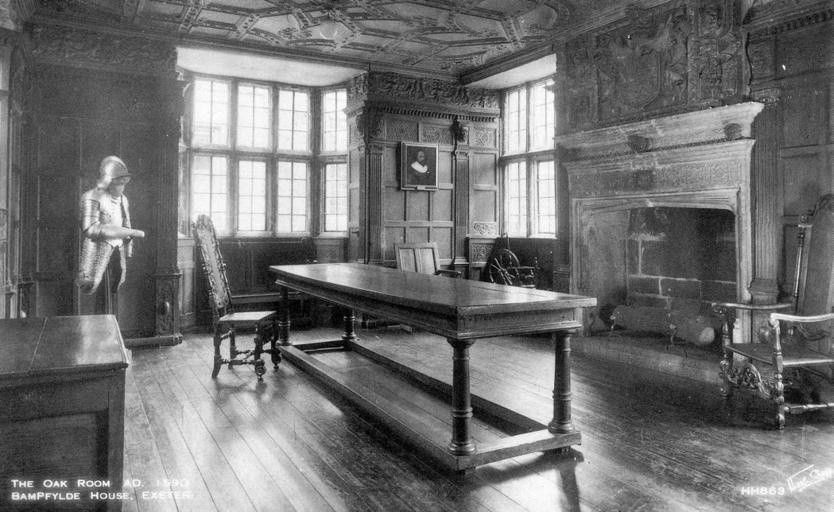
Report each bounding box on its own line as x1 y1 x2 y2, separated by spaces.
408 150 432 185
74 155 145 295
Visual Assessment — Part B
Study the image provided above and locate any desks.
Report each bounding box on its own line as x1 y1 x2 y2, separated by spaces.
266 261 598 484
0 312 129 512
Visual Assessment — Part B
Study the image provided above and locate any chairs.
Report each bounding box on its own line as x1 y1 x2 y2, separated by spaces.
706 192 833 432
191 213 283 386
394 241 461 278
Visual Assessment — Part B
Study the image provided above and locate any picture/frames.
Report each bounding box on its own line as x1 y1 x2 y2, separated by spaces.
399 141 439 192
